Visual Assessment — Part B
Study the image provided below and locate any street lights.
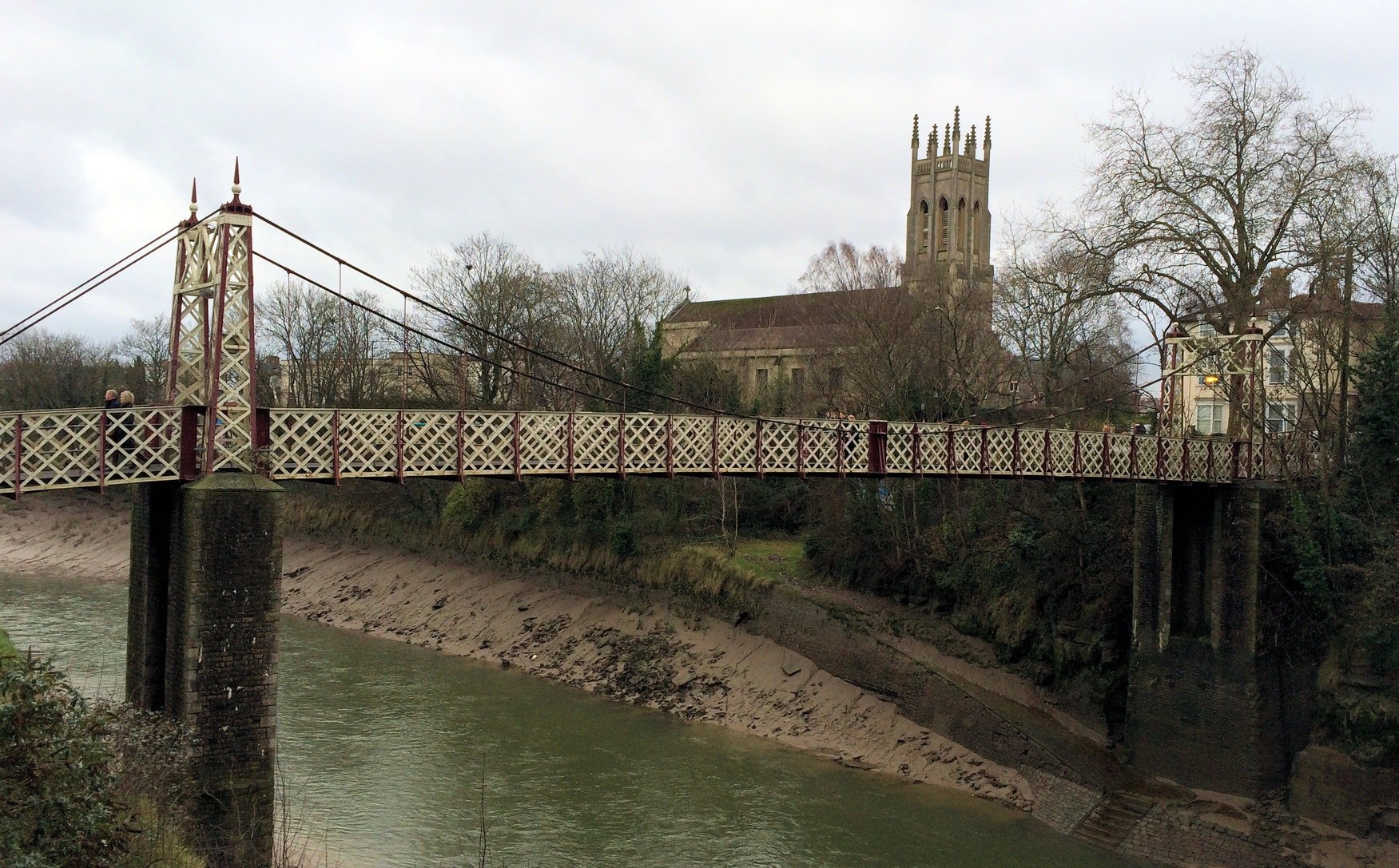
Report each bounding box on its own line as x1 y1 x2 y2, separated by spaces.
1239 304 1264 441
1165 312 1185 437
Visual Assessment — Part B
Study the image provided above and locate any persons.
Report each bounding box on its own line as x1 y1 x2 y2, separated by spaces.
958 421 973 465
836 411 860 469
1137 423 1146 434
94 388 138 471
1102 422 1109 434
